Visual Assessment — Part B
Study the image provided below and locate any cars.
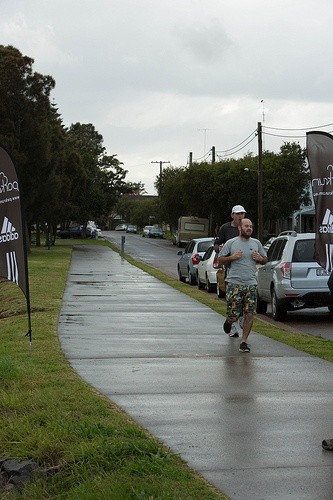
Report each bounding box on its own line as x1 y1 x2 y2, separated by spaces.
216 246 270 299
125 225 137 234
196 245 226 293
115 224 127 231
143 225 163 239
56 223 102 239
176 236 216 285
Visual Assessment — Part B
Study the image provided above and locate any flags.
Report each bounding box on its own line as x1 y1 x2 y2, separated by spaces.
0 144 28 297
306 131 333 273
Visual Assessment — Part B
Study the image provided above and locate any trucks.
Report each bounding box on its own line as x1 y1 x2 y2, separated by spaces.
172 216 209 247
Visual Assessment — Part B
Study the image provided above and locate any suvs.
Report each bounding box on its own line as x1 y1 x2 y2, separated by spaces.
256 229 333 322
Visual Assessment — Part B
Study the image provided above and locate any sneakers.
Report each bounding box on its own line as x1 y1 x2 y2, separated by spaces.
239 317 245 329
239 342 250 352
224 319 231 334
230 325 239 337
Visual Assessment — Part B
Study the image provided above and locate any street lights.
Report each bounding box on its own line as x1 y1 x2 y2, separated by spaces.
243 167 263 244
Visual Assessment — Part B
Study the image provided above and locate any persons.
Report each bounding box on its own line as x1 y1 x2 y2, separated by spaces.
218 205 247 337
217 218 268 352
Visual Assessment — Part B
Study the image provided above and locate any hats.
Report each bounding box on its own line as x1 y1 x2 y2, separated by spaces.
232 205 246 213
